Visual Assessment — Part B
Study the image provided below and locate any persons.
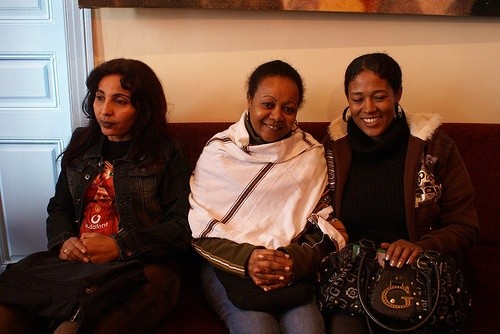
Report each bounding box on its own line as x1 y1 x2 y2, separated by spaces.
187 60 327 334
321 53 481 334
0 58 192 334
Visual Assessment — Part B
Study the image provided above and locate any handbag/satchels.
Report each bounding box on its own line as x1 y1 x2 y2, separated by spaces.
315 239 477 333
0 249 146 324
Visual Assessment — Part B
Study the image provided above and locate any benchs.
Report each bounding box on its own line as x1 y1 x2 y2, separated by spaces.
157 122 500 333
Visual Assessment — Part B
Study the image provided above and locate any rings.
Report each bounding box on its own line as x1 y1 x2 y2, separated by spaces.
63 248 68 253
